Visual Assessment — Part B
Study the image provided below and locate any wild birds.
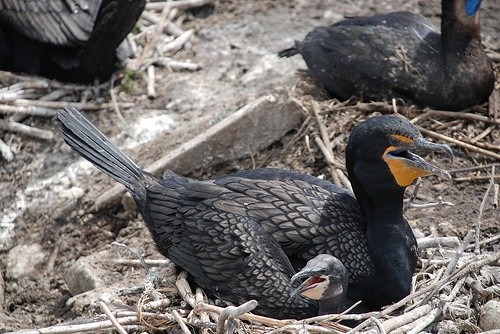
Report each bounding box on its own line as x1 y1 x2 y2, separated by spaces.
55 104 454 320
0 0 147 85
277 0 495 110
